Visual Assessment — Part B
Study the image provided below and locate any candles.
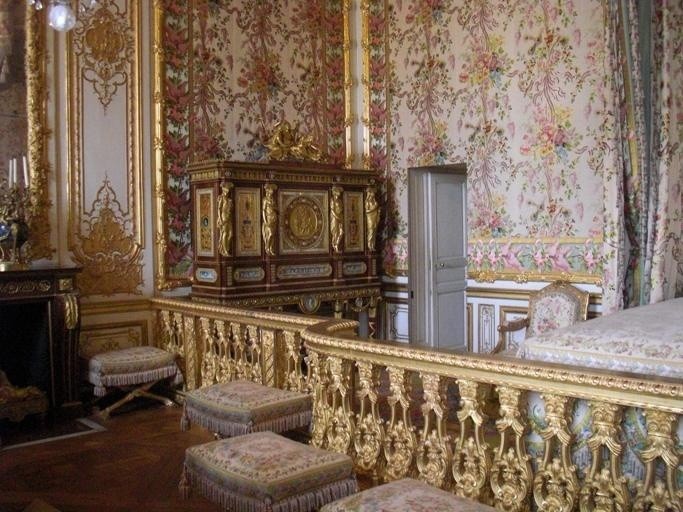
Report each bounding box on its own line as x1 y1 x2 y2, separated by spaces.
7 154 28 189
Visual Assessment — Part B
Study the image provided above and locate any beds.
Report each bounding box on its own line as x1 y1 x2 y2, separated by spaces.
522 296 683 484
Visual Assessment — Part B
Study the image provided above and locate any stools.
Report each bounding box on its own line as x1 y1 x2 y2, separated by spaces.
78 344 177 421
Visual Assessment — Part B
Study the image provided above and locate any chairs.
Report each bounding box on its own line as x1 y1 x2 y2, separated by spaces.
485 278 591 354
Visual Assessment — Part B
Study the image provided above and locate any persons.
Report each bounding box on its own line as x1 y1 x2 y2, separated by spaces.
261 182 280 257
215 182 234 257
328 185 345 253
269 109 293 161
365 186 383 253
